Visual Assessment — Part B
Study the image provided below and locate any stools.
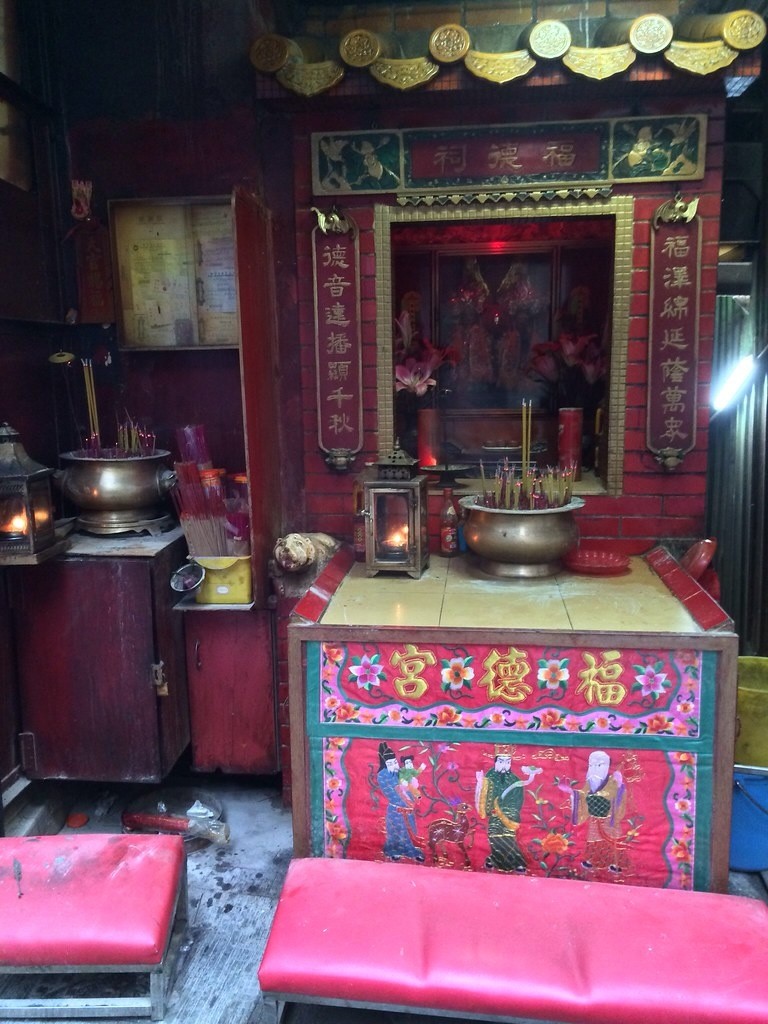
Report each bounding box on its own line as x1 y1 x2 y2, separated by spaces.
0 833 189 1019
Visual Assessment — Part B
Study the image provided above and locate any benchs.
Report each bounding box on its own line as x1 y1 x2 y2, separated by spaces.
258 857 768 1024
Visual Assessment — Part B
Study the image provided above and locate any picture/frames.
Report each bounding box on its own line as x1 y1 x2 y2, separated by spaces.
428 245 561 419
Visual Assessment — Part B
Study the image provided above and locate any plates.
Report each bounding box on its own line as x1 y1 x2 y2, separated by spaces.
481 444 522 451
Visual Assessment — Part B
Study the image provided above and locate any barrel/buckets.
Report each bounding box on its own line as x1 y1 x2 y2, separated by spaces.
728 655 768 874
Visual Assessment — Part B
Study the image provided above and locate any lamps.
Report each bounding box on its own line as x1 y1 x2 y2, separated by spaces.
361 438 430 579
0 420 71 566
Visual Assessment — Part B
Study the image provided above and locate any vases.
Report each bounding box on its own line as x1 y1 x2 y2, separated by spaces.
400 409 417 451
549 402 596 469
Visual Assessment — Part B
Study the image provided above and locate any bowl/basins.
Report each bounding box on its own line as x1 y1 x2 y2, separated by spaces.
678 534 718 580
561 550 631 575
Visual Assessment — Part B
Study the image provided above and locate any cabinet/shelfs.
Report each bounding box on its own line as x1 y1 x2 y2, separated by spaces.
181 609 281 775
11 517 191 786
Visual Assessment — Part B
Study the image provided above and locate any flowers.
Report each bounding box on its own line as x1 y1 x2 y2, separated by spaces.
394 309 454 409
523 331 608 403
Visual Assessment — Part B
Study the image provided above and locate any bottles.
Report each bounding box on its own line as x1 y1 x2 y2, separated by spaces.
438 488 458 559
